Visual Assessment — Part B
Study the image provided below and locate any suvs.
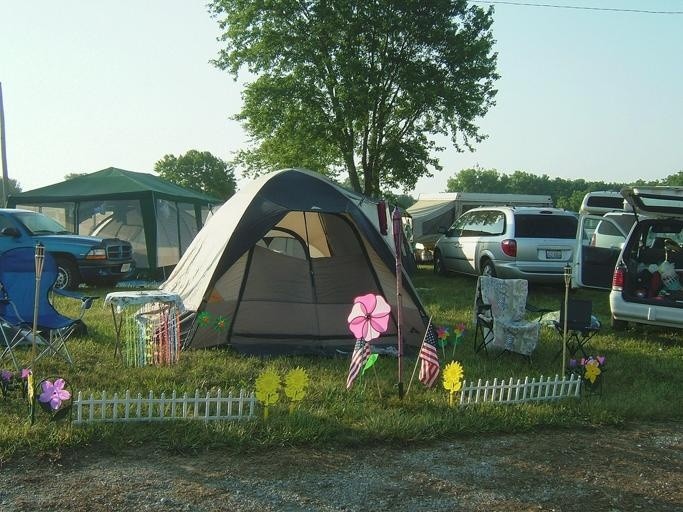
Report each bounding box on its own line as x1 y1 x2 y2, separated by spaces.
0 208 136 288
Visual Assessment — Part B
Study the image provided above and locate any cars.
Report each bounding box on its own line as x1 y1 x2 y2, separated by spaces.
570 185 683 331
431 206 590 287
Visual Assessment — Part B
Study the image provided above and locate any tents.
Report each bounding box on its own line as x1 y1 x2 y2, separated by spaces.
123 167 442 355
400 187 555 253
3 165 227 290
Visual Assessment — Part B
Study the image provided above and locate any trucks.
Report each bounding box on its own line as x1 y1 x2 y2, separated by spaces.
411 192 554 265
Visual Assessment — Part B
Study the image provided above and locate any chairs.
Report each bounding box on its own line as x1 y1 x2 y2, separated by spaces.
0 246 100 376
549 298 602 368
474 275 553 366
647 237 673 258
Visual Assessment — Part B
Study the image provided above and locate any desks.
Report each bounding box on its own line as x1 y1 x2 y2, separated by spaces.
106 290 180 367
